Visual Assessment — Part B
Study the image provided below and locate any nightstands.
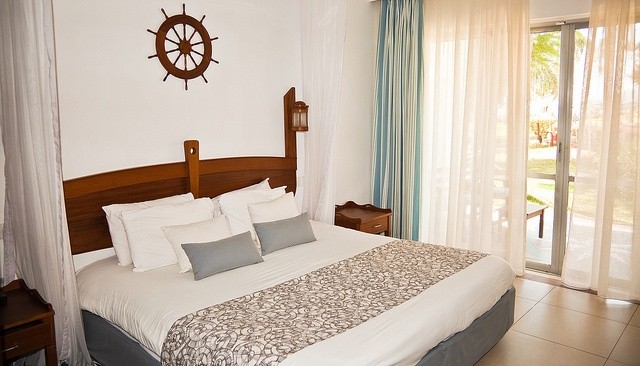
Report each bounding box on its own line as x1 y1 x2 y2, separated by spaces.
334 200 392 238
0 278 58 366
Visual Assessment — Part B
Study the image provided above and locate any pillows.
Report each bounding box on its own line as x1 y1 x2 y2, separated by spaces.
115 196 214 273
181 231 264 281
161 214 233 274
219 185 288 236
252 212 318 257
248 191 302 247
211 177 271 217
101 192 195 268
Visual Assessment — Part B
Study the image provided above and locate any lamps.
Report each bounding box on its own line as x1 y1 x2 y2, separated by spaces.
289 100 310 133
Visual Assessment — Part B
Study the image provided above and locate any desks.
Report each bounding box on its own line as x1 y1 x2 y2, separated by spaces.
526 204 549 240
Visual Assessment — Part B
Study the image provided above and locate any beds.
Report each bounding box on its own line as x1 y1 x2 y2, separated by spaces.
63 139 518 366
